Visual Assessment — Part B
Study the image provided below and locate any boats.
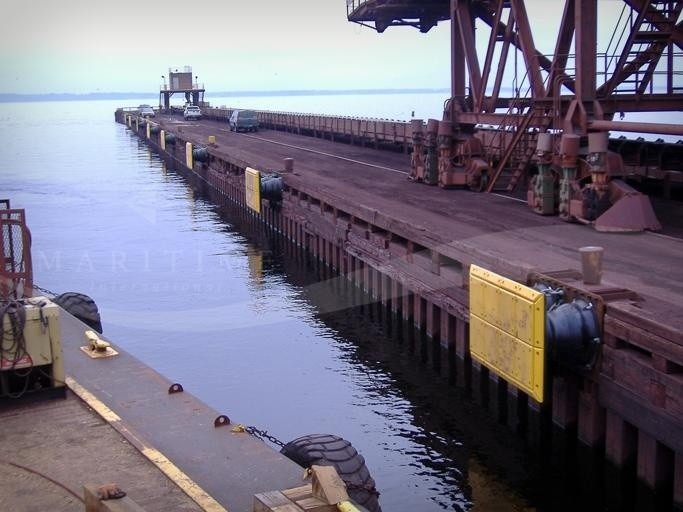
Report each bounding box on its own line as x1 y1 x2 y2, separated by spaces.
0 193 383 511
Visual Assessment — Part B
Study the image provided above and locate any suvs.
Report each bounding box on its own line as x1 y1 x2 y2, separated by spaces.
228 109 261 133
183 106 202 121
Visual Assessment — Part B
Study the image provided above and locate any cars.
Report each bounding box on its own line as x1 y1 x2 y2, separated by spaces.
139 107 155 118
